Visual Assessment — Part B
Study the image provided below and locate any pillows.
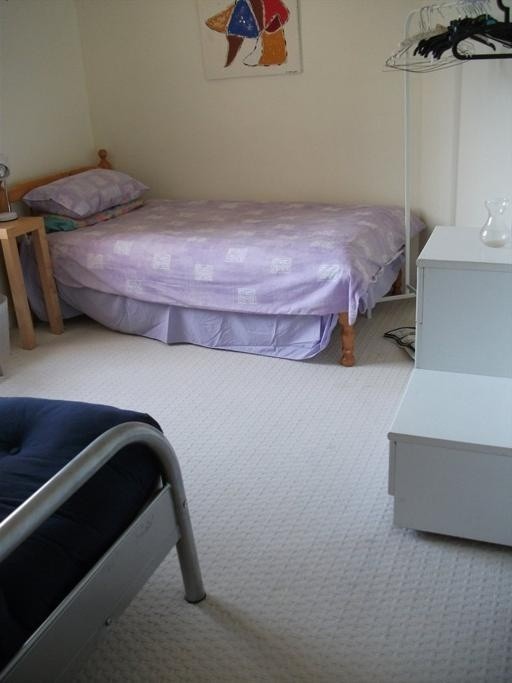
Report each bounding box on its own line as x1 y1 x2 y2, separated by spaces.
23 167 149 217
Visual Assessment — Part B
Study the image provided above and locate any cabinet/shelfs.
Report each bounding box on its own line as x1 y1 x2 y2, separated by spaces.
385 229 512 547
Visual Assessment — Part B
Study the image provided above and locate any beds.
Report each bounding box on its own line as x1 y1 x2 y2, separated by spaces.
0 149 424 364
0 398 207 682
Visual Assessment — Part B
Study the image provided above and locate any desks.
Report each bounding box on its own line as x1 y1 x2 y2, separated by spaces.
1 216 63 349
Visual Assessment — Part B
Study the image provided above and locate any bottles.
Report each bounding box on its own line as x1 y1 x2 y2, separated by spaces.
480 198 510 248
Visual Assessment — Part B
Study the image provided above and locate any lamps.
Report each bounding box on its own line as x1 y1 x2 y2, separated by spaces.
0 162 15 221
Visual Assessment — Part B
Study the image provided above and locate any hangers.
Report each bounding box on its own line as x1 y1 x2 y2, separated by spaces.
380 0 512 73
